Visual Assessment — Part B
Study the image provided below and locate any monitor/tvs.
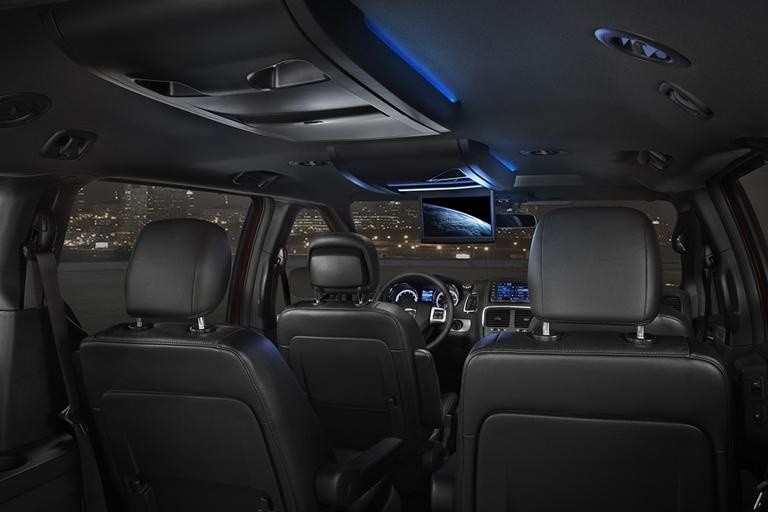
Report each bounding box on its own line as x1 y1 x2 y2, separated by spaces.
418 188 496 244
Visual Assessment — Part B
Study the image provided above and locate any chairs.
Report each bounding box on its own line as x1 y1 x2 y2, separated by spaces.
274 233 458 465
529 312 689 336
450 209 733 510
78 220 405 510
